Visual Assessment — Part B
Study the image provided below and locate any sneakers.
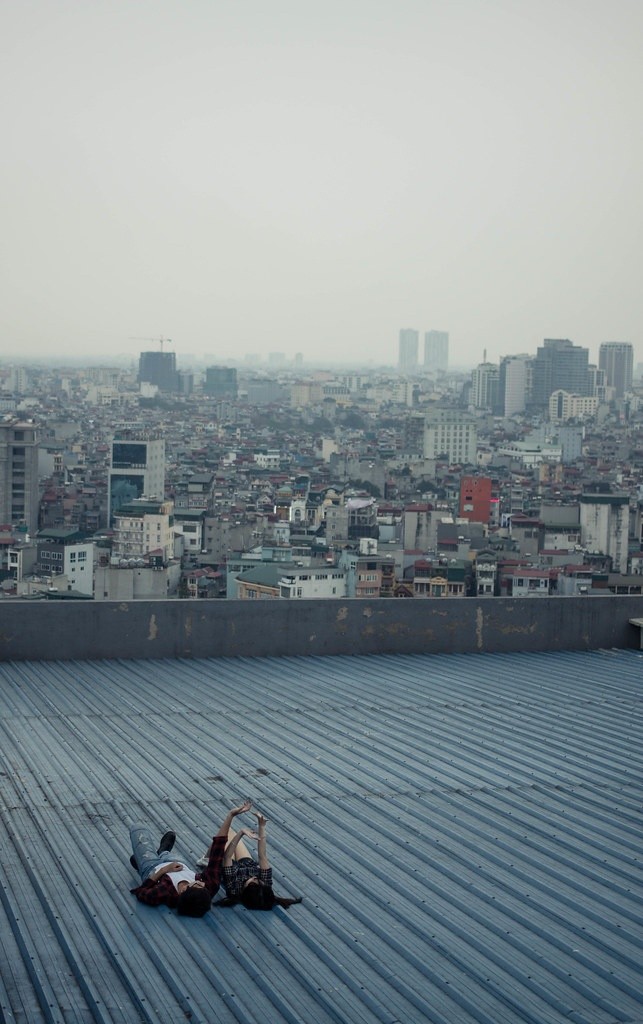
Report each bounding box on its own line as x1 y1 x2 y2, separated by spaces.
196 856 209 866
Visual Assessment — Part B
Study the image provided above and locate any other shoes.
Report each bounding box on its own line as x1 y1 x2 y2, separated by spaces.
157 831 175 855
130 855 139 871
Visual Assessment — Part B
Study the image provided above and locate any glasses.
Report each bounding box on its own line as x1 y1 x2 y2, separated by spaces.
191 882 204 888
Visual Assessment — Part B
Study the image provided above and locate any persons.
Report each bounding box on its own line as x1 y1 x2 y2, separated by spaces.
213 811 302 911
129 798 252 918
